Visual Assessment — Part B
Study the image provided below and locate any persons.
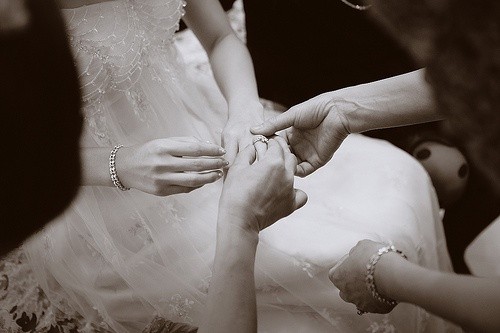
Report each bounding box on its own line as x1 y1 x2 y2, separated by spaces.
250 0 499 332
24 0 443 332
1 2 307 333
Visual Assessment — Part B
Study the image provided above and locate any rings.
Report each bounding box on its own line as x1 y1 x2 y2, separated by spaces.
356 308 367 315
253 136 268 146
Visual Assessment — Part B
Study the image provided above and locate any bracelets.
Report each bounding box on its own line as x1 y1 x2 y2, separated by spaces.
367 246 407 305
110 144 130 191
